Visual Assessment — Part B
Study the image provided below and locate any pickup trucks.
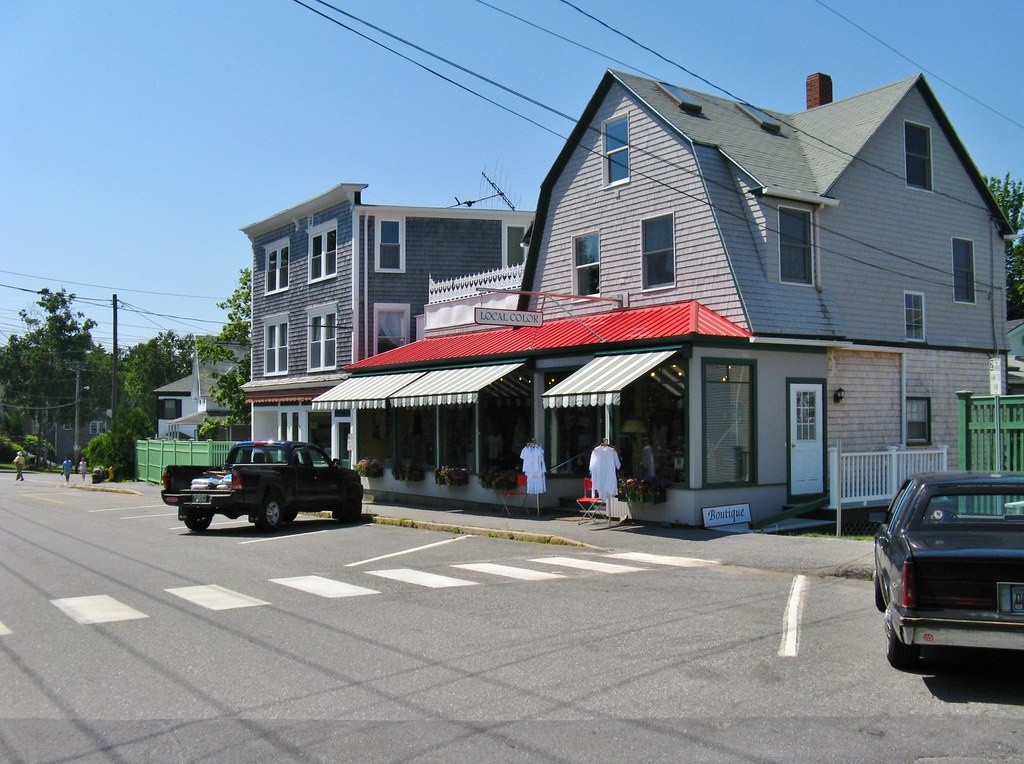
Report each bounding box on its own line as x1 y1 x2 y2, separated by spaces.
161 440 368 534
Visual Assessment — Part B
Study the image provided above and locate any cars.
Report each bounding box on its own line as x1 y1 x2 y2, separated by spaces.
869 470 1023 667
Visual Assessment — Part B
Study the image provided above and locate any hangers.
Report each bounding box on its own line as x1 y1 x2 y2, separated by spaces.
595 438 614 450
522 438 544 452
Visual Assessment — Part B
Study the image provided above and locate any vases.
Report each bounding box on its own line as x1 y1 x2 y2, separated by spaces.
617 490 667 505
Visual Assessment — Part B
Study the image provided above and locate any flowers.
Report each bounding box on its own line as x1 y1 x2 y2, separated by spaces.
352 458 518 492
618 479 666 508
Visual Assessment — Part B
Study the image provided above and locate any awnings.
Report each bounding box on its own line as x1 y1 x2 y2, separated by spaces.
539 347 685 407
310 360 428 411
390 362 526 408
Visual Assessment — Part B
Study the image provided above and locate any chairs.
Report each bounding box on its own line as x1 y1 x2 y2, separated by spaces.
923 498 956 522
500 474 530 518
253 453 274 463
284 453 305 466
576 478 607 525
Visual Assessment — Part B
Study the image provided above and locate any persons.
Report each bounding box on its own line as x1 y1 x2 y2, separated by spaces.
79 457 88 482
61 455 72 482
12 450 26 481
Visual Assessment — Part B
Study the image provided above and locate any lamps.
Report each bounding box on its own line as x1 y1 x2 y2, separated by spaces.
833 387 845 402
